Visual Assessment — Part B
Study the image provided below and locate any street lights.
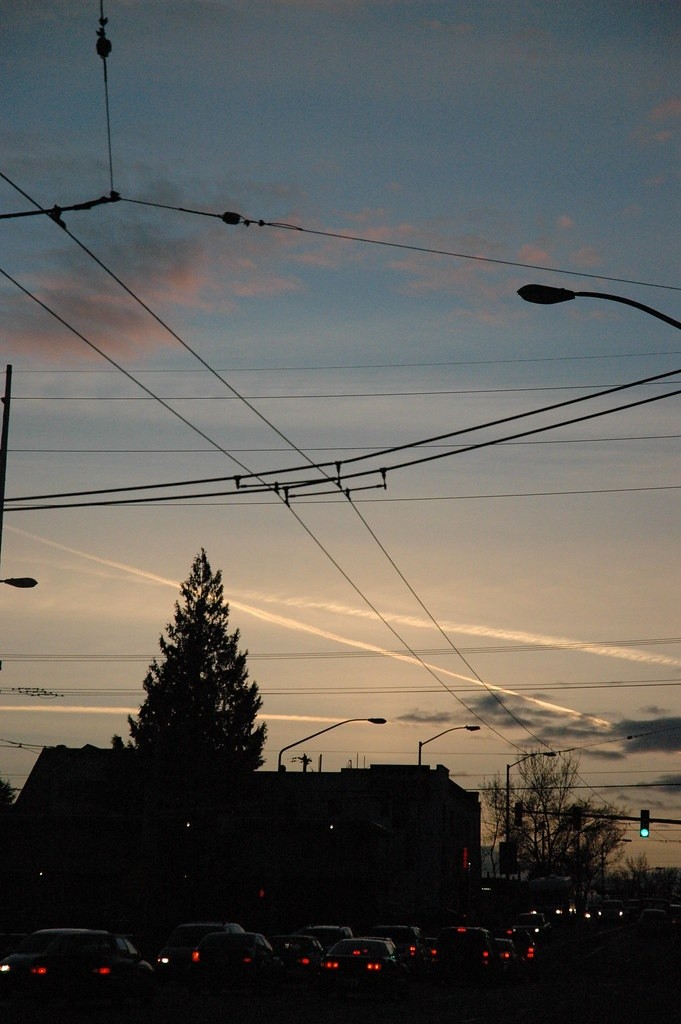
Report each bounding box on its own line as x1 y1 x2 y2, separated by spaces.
278 718 390 779
418 725 481 770
505 751 559 841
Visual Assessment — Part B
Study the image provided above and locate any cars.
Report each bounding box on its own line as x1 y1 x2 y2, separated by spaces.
0 927 115 1003
153 921 247 982
184 932 288 995
317 936 413 1001
422 893 681 982
367 925 429 978
291 925 355 954
269 932 324 984
29 931 156 1006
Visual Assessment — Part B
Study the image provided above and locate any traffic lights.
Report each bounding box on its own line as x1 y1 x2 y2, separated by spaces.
640 810 650 838
